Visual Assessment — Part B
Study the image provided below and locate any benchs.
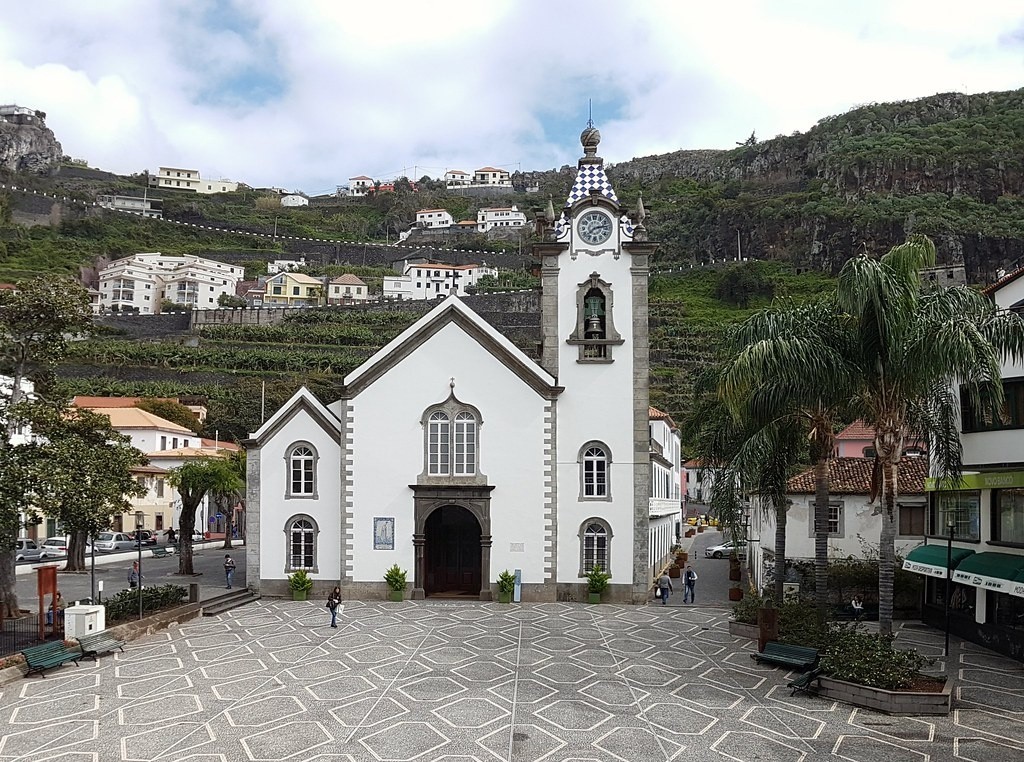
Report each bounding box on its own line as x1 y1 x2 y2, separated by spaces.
21 640 83 678
752 641 823 699
76 629 125 662
174 543 194 554
150 547 173 558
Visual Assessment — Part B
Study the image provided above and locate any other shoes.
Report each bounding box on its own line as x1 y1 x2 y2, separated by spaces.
683 600 686 604
331 624 337 628
226 585 231 589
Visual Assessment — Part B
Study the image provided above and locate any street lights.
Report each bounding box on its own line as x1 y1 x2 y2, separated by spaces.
945 508 956 657
135 511 145 620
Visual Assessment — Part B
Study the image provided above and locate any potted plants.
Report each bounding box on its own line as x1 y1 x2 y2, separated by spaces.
698 525 703 532
728 580 743 600
584 564 611 604
383 562 408 602
496 569 517 603
669 548 688 578
685 527 695 538
288 570 313 601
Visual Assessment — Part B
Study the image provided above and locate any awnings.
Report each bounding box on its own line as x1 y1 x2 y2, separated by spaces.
1008 566 1024 598
952 552 1024 594
901 543 975 579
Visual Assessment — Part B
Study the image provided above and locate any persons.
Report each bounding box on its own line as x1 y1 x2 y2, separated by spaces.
127 560 146 587
162 527 176 541
696 513 700 520
682 565 698 604
659 571 674 605
44 592 66 627
696 518 702 526
705 512 711 526
327 586 342 627
223 554 236 589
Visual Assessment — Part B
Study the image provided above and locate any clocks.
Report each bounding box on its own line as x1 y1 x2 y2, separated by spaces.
577 211 612 245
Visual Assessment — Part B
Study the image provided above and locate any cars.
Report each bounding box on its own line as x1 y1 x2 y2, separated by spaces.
687 515 718 526
705 539 748 559
16 529 206 561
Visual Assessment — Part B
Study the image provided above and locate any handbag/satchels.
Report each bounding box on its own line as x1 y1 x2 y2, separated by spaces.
656 587 661 597
336 604 344 614
326 592 335 609
